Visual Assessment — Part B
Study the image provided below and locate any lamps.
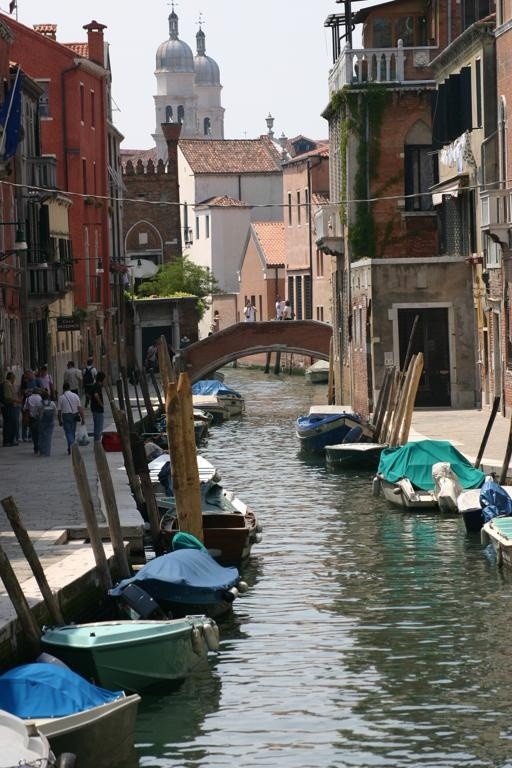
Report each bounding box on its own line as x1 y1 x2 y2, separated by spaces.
1 219 32 250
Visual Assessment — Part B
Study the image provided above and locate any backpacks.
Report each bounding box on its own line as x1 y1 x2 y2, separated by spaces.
41 400 55 422
83 367 95 384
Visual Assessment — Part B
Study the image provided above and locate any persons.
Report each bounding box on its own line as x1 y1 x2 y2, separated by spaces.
243 299 257 322
162 461 174 497
146 340 158 372
275 294 292 320
180 336 190 349
0 357 105 454
212 310 220 333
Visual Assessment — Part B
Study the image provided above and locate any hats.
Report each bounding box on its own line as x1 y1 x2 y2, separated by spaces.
181 336 190 342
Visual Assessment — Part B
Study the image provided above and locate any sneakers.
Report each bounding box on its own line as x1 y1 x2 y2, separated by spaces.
34 449 50 456
4 438 33 447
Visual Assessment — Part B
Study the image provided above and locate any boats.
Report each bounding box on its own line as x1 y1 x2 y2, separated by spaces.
294 404 374 452
138 453 222 498
192 407 214 437
323 441 389 474
41 611 223 701
456 476 512 532
479 514 512 574
154 419 207 447
191 378 245 422
155 485 264 565
304 358 330 383
371 440 491 509
0 653 144 753
108 548 248 622
0 709 78 767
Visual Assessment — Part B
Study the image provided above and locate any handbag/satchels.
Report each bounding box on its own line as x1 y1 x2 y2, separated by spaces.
75 415 81 422
